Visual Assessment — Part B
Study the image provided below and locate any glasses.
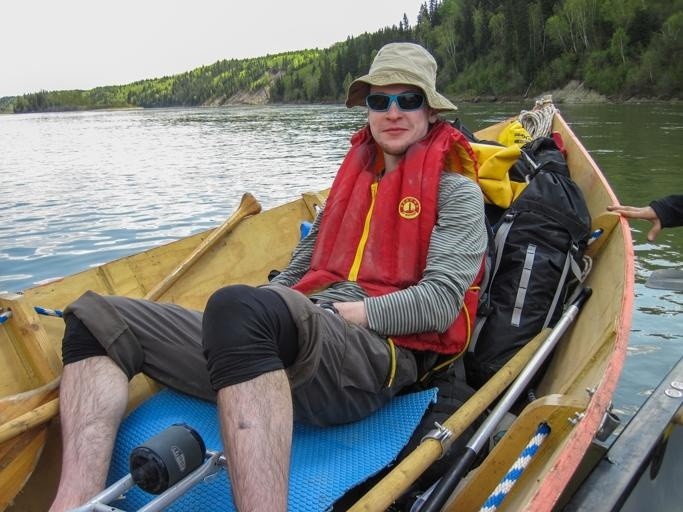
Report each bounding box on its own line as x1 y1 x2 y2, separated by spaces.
364 90 425 112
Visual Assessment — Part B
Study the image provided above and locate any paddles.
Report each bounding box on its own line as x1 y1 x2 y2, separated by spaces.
1 192 261 512
645 269 682 290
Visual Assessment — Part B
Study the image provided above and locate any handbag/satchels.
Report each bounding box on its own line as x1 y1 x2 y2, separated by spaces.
453 137 591 411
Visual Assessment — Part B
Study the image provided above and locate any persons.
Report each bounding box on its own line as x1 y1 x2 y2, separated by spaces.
46 43 490 512
604 192 683 241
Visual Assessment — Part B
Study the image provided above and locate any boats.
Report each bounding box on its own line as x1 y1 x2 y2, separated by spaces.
0 94 635 512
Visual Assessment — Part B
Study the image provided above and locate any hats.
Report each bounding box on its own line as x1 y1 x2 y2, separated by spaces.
346 42 459 114
